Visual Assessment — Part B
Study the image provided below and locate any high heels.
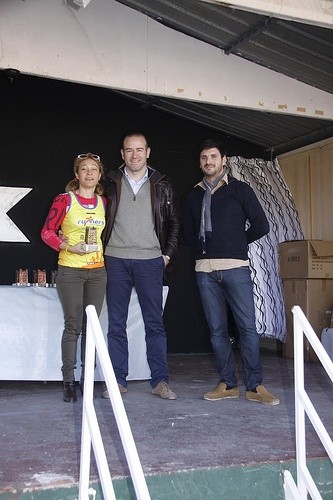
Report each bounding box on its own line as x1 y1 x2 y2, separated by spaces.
62 381 77 403
79 386 96 399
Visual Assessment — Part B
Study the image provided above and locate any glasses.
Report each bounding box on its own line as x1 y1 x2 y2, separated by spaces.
78 154 100 162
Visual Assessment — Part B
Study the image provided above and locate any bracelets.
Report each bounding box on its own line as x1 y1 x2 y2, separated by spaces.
66 244 73 254
166 255 170 259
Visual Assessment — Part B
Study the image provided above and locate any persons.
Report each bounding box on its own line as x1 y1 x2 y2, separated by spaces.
96 129 180 400
184 140 280 405
40 152 109 402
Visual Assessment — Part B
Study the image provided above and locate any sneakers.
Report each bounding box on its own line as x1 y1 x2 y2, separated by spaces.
102 383 128 398
151 380 177 399
203 382 239 401
246 385 280 405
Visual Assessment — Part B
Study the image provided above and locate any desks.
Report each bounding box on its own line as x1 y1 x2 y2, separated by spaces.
0 285 169 384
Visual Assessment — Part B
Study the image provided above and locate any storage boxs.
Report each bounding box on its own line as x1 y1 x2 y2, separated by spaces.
279 240 333 279
281 278 333 362
